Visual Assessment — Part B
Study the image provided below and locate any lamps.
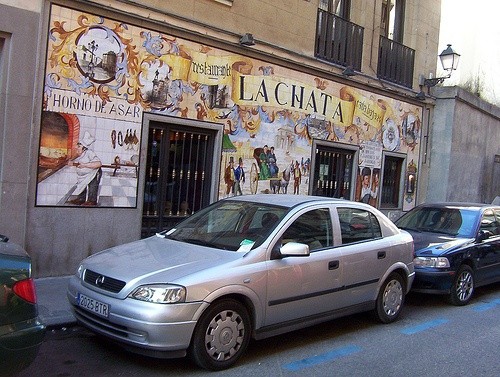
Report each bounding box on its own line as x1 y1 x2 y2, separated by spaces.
341 64 356 78
422 42 461 94
240 31 256 46
417 73 427 100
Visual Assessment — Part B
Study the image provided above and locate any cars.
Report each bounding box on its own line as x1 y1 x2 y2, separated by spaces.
0 234 47 375
67 192 416 371
393 199 500 306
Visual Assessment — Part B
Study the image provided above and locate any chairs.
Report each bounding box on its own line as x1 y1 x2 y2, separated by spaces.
428 212 486 241
246 211 369 254
